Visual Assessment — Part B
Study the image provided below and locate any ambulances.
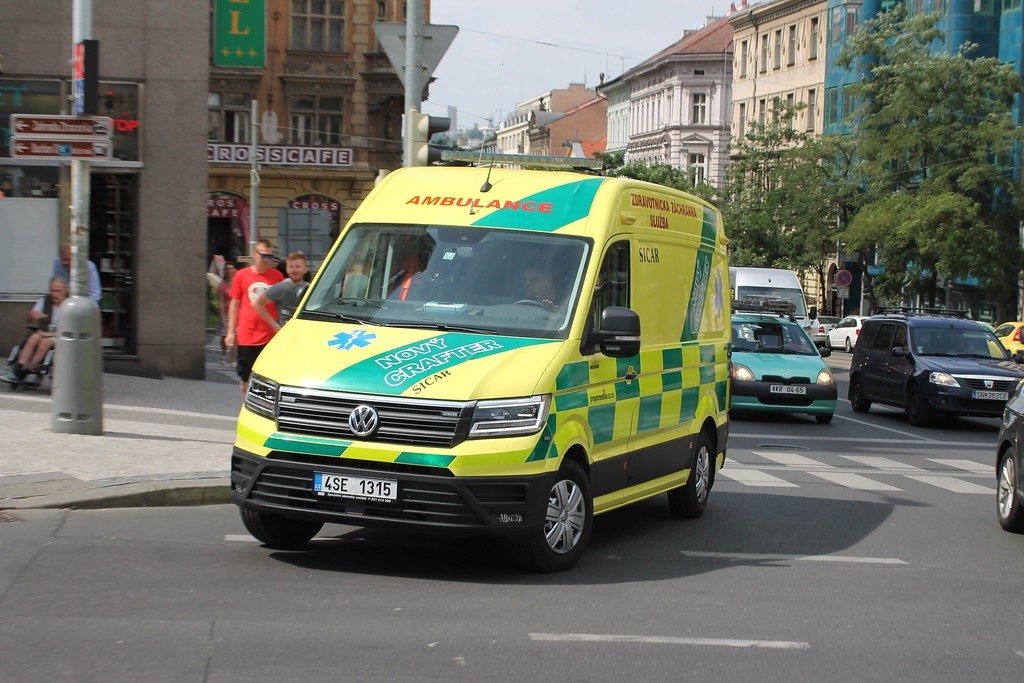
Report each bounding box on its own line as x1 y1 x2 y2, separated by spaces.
228 142 735 575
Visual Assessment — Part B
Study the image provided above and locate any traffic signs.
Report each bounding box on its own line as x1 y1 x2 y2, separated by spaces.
8 115 112 163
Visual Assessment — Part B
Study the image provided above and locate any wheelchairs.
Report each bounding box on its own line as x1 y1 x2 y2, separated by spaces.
5 324 55 390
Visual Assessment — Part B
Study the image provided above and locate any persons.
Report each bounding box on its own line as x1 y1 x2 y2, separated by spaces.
8 276 70 384
208 237 383 402
53 241 102 303
518 257 590 350
945 335 977 354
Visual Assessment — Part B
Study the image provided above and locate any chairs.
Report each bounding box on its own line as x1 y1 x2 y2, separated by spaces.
548 243 584 302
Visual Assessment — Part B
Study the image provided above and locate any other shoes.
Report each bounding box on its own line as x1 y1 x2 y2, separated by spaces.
21 373 41 388
231 362 238 369
0 371 20 384
220 355 227 365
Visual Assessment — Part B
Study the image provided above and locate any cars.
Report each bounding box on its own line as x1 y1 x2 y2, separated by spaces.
986 318 1024 359
729 312 839 422
826 315 872 351
809 315 840 344
996 370 1024 530
848 311 1024 426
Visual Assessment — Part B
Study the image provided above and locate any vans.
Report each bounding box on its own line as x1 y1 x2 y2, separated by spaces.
728 267 818 344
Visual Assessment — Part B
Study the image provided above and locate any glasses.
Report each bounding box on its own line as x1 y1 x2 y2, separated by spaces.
257 252 275 260
521 276 537 284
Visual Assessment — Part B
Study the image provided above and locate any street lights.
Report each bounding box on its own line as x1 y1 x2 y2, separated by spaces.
407 111 452 167
